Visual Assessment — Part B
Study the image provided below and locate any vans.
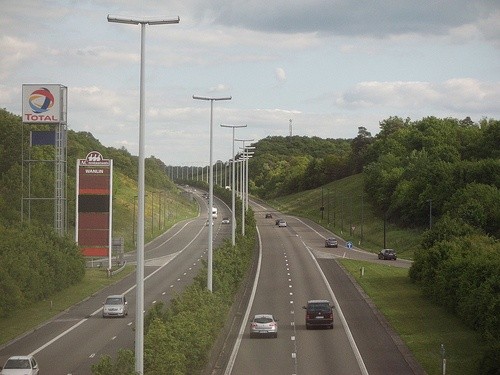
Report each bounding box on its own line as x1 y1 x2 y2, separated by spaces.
207 207 218 219
303 300 336 330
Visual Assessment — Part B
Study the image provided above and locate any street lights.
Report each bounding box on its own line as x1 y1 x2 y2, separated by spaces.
191 94 232 294
224 139 256 237
106 14 180 375
219 123 247 247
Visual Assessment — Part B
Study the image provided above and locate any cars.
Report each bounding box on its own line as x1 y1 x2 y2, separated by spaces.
276 218 287 227
249 314 280 338
206 220 215 226
266 213 272 218
0 355 40 375
102 295 128 317
200 192 210 200
325 237 338 248
221 216 230 224
377 248 397 261
